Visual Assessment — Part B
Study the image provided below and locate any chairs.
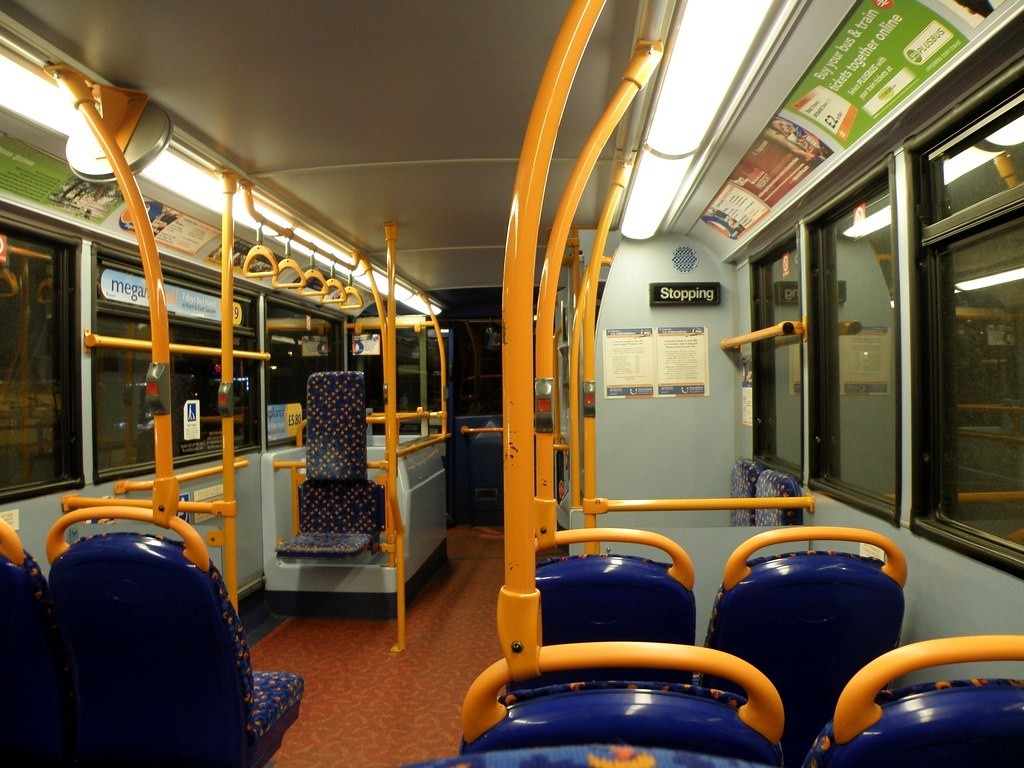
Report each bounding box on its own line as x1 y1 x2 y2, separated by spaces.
45 504 305 768
0 517 55 768
274 372 378 559
691 521 908 768
754 469 804 528
532 526 696 685
727 454 763 529
457 640 788 768
802 635 1024 768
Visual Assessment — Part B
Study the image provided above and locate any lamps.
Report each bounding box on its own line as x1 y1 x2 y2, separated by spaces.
0 49 448 325
614 0 775 239
